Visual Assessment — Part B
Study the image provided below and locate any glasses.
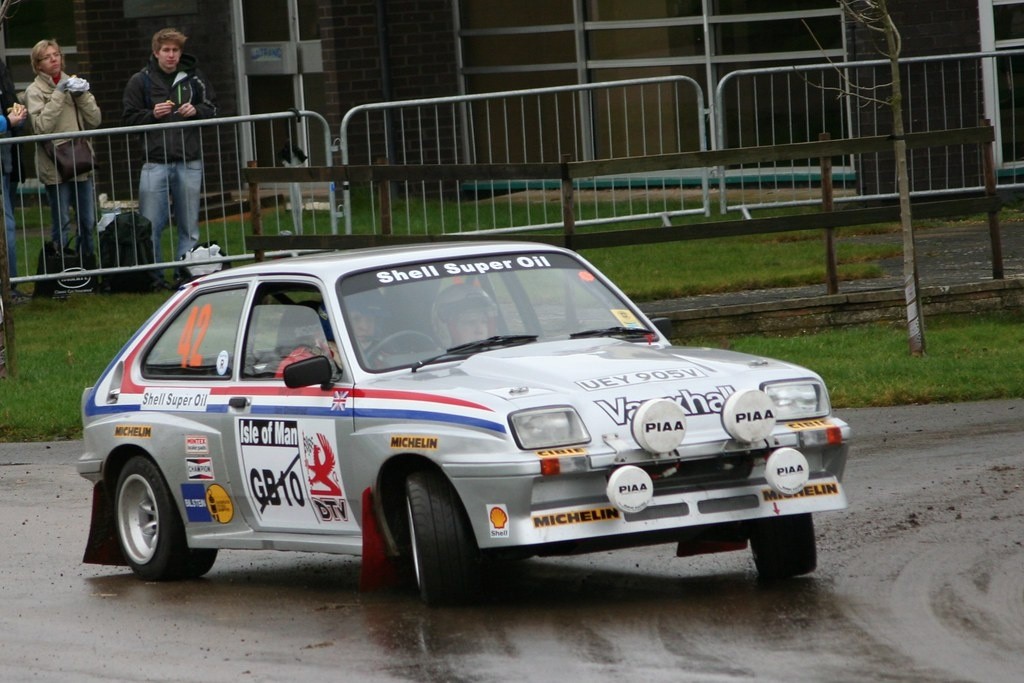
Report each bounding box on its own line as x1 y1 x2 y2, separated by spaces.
39 53 62 62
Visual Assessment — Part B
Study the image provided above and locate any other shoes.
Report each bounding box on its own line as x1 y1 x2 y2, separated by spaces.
11 293 33 304
152 277 164 294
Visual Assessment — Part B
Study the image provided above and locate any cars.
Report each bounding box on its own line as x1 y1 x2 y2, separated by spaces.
75 244 850 610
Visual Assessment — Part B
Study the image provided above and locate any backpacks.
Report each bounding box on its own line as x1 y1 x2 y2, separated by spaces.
96 210 160 294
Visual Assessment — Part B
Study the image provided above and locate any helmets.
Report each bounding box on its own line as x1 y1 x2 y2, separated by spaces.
316 287 389 342
429 284 498 349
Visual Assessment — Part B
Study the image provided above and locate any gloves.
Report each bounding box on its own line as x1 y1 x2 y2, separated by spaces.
56 79 69 94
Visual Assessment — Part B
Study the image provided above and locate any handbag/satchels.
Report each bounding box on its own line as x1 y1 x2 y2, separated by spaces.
175 240 231 290
32 217 98 300
52 138 102 184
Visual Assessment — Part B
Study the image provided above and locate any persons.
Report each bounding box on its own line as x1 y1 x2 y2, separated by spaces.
275 286 390 379
432 283 497 349
23 40 101 255
121 29 221 281
0 60 33 305
